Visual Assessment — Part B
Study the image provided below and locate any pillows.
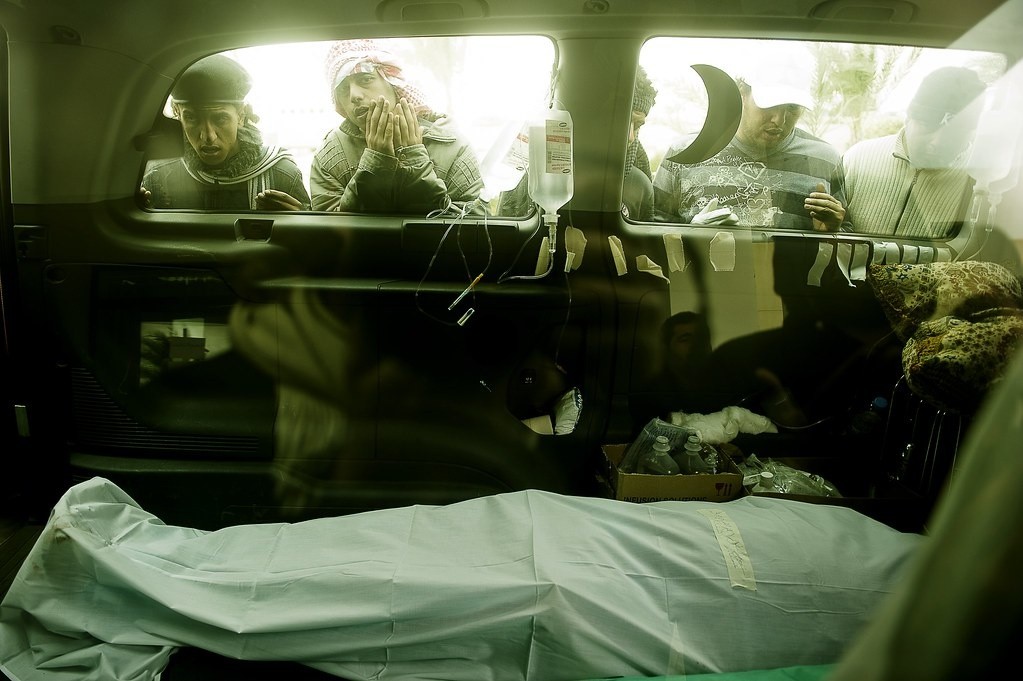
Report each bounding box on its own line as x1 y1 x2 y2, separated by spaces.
867 260 1023 407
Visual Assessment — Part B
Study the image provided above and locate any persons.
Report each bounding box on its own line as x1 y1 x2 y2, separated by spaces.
646 312 751 455
310 36 492 217
492 53 657 219
653 39 854 235
137 53 311 211
839 66 986 239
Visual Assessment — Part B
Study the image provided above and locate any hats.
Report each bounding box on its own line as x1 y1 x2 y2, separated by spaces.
741 47 815 112
170 52 249 104
631 64 654 116
908 65 986 133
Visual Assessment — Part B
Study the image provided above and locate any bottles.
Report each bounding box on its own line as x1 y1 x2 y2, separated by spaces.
636 435 680 476
527 105 575 227
675 435 708 475
751 470 778 493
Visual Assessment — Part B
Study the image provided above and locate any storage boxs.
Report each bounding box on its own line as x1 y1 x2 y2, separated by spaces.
598 445 741 503
743 457 847 506
